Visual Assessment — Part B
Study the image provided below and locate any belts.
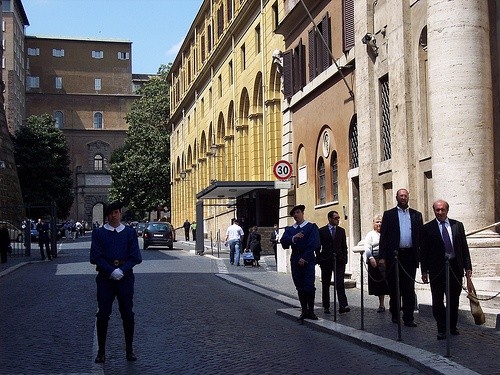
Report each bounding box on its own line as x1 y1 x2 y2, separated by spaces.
114 260 123 266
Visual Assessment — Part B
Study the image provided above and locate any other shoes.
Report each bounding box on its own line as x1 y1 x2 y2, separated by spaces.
376 306 385 312
392 318 398 323
451 331 459 335
297 312 307 320
308 313 318 320
324 310 331 314
404 320 417 327
339 308 350 314
437 332 445 338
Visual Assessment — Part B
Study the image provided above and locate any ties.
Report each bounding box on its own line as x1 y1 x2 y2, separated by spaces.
440 221 453 254
331 228 335 239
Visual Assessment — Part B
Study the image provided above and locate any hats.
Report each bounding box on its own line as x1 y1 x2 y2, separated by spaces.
290 205 305 216
105 203 121 212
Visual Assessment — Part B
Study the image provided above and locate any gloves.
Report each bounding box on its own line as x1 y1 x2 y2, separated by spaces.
109 268 124 280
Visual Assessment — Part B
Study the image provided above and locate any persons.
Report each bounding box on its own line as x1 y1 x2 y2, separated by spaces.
319 210 351 314
182 219 196 241
245 224 278 268
224 220 244 267
366 215 388 310
280 206 320 322
63 218 87 241
419 200 472 340
379 189 426 327
90 204 143 365
36 217 53 261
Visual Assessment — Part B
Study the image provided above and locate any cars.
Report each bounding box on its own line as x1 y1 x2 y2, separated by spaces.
0 221 23 243
143 222 174 250
136 223 144 236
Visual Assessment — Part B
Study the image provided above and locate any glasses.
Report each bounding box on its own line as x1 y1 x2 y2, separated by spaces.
331 217 340 220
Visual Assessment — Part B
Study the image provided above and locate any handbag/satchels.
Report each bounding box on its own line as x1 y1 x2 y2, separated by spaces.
465 273 485 325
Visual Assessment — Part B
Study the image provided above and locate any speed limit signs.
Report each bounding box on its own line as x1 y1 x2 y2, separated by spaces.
273 160 293 180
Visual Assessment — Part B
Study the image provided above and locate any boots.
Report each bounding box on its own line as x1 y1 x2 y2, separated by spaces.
123 319 136 360
95 320 108 362
